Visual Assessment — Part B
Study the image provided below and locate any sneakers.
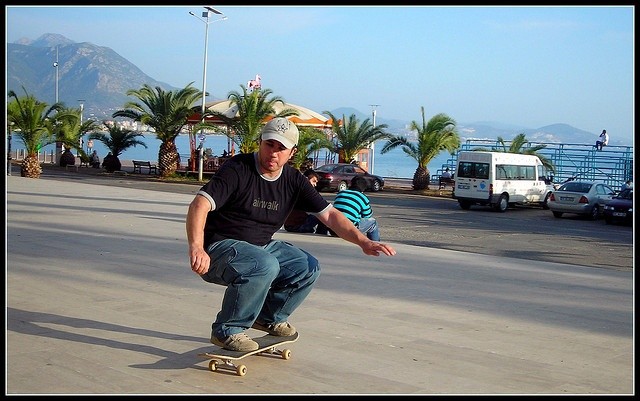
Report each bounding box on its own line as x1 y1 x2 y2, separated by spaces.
251 320 296 337
211 330 259 353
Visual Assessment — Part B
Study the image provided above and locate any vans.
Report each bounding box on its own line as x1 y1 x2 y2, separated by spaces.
455 149 556 212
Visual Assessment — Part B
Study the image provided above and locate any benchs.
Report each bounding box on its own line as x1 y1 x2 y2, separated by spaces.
80 157 100 169
438 177 455 191
132 161 157 175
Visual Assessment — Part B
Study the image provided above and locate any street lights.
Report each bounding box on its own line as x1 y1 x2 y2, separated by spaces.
78 98 86 155
369 102 380 173
187 6 229 179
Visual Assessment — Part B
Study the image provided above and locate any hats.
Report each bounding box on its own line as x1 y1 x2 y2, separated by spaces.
261 117 299 150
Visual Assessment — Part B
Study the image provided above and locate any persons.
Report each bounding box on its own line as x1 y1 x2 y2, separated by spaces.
89 149 100 167
596 129 609 151
442 167 451 177
284 168 328 234
186 116 397 352
102 151 113 167
328 175 380 241
621 180 633 192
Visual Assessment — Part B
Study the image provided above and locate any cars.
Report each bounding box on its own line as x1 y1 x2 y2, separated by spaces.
547 180 617 219
604 188 633 225
313 162 384 191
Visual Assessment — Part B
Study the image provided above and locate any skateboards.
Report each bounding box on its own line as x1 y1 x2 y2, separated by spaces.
197 331 299 376
